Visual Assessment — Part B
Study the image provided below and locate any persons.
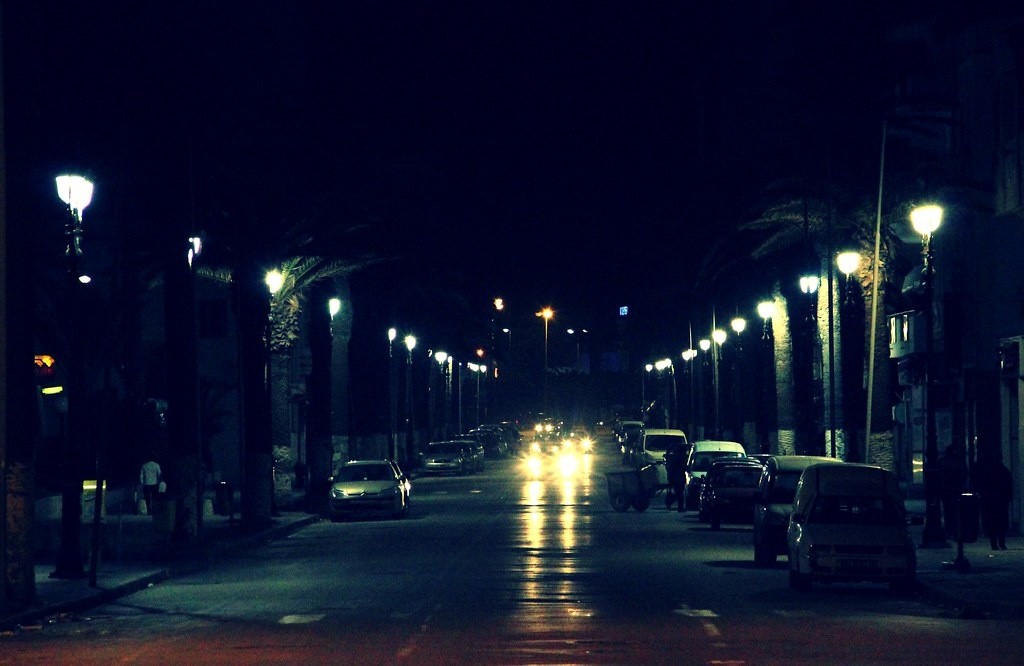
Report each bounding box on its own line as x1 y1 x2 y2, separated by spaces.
141 461 161 516
986 456 1012 552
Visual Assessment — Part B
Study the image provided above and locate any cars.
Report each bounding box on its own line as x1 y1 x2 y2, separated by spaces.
417 440 464 475
678 440 748 515
609 419 644 449
452 433 485 473
327 458 404 521
753 455 846 563
630 426 688 490
699 456 764 529
785 461 915 602
468 428 507 460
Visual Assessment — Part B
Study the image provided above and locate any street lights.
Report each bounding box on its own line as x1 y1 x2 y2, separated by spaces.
911 204 951 546
644 274 821 456
388 326 490 465
185 233 207 561
45 173 92 578
835 250 862 463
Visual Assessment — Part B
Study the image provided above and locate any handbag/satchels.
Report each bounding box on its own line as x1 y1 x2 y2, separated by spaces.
666 485 674 505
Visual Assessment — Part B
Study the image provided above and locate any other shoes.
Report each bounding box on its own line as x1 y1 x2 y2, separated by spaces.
678 509 686 512
991 545 1007 550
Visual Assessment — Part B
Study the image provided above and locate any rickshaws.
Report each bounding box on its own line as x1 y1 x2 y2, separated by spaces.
604 463 672 515
